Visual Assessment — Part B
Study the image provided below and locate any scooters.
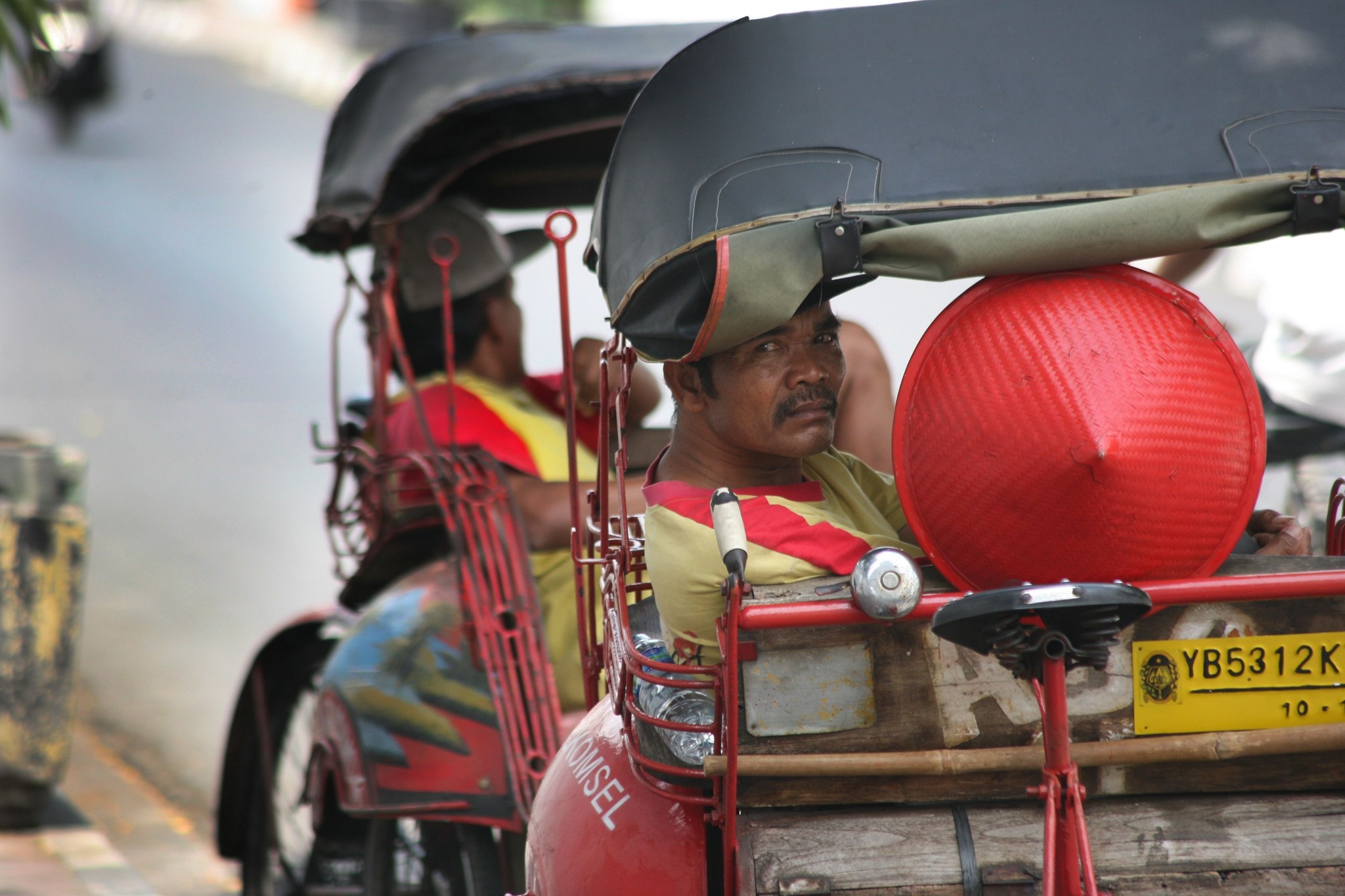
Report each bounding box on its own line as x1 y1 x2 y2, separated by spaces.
24 0 108 140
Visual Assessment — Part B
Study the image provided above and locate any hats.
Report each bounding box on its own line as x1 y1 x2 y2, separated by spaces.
373 200 548 309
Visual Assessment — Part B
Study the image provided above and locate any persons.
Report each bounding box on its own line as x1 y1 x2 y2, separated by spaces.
384 200 892 710
641 299 1313 670
1150 231 1344 454
